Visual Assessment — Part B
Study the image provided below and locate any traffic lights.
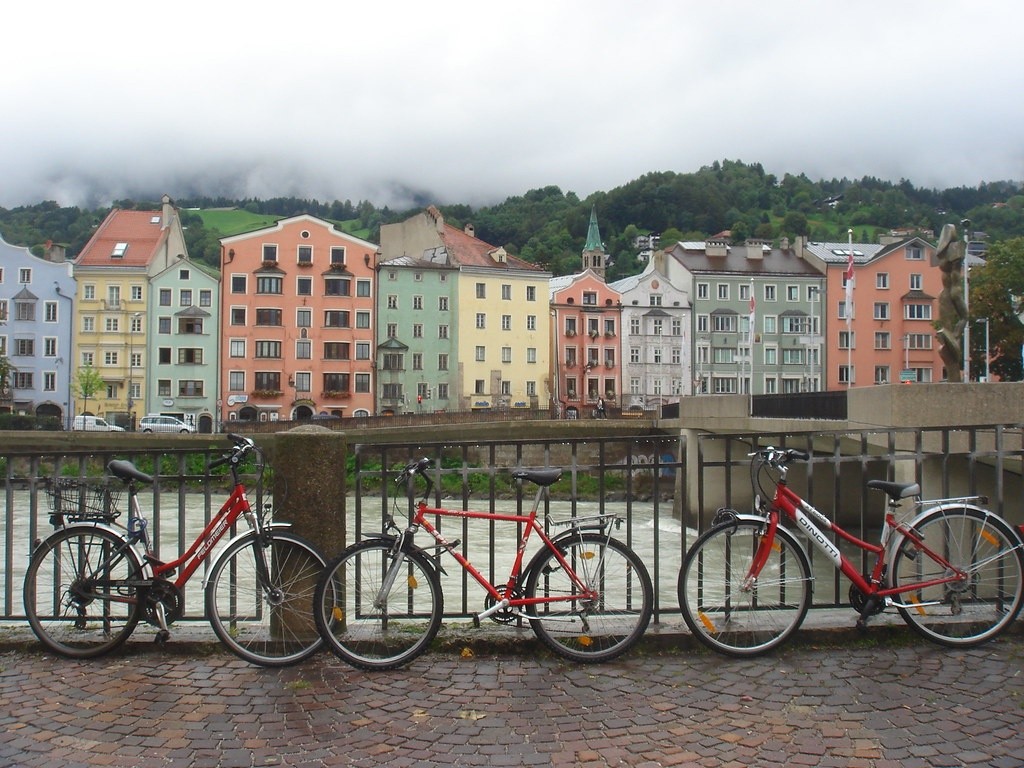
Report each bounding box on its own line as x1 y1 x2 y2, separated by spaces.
418 396 421 404
427 389 431 400
401 395 404 404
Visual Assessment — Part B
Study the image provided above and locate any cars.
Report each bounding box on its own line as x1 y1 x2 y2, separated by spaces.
311 415 340 419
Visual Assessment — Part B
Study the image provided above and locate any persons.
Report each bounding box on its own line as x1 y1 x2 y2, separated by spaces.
596 396 607 421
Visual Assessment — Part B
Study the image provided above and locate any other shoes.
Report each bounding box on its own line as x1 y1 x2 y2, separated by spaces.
596 418 602 421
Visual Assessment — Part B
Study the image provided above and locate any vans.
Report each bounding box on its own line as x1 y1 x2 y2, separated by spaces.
73 416 125 432
138 416 197 433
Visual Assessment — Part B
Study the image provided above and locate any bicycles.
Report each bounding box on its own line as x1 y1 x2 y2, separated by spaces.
677 445 1024 657
312 457 653 670
23 432 342 667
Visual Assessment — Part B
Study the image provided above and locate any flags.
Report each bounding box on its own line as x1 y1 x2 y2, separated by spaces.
844 240 854 326
746 284 755 337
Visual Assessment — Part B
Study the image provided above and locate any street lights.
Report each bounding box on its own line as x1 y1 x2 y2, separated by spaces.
960 219 971 383
899 338 909 370
976 317 989 382
